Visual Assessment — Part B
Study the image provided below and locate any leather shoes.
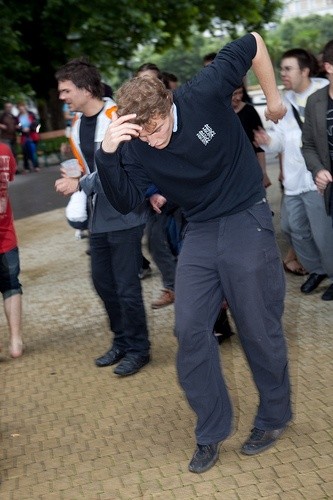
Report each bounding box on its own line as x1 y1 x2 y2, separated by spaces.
301 273 327 293
321 283 333 300
113 352 149 375
95 347 126 367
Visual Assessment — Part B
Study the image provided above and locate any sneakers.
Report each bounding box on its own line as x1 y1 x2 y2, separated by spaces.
189 435 224 473
241 426 284 454
151 288 175 308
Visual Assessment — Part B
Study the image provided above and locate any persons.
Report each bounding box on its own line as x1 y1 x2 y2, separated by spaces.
0 101 22 176
0 138 26 363
64 188 90 240
55 59 152 377
300 38 333 219
88 47 330 349
253 48 333 301
95 29 292 473
60 103 78 154
13 101 42 174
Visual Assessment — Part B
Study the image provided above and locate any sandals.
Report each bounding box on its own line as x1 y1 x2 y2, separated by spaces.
283 258 306 276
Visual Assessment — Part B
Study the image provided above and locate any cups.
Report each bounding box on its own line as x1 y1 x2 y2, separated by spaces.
61 159 82 178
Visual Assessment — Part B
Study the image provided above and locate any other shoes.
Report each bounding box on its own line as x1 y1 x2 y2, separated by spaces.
210 307 231 343
137 267 152 279
34 167 40 171
15 170 20 175
24 169 30 173
